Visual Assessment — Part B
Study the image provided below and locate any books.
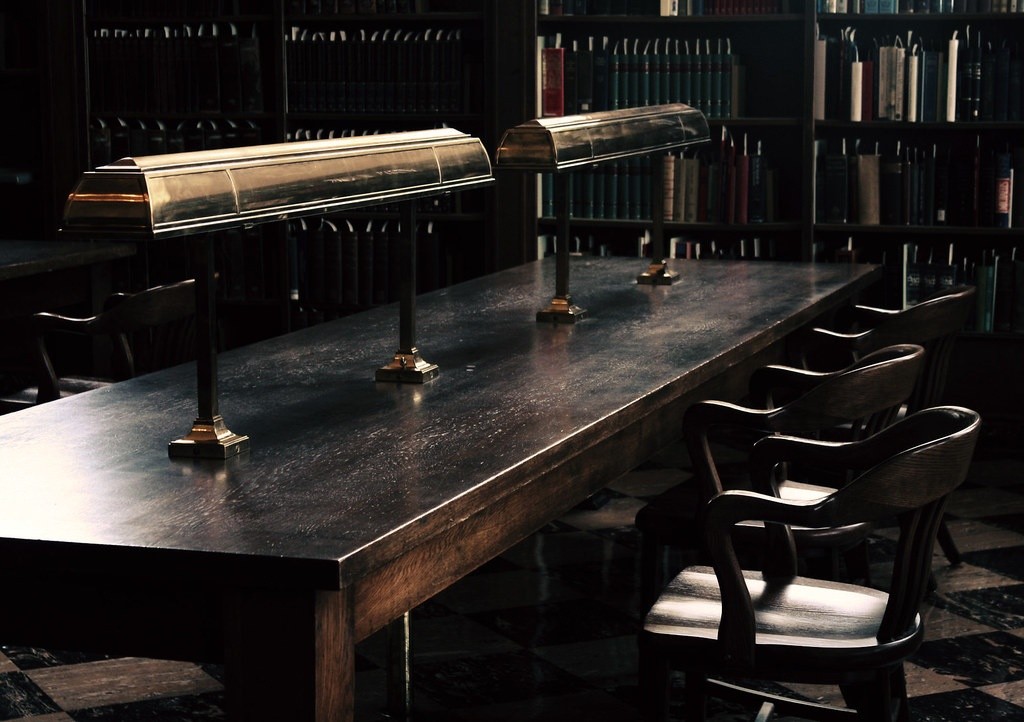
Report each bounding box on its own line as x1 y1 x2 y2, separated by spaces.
534 34 781 260
87 0 264 378
815 37 1024 334
538 0 1024 17
285 0 463 334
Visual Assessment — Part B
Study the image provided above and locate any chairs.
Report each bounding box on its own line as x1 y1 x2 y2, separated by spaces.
0 643 239 722
0 271 221 414
633 342 927 677
638 404 981 722
758 281 976 567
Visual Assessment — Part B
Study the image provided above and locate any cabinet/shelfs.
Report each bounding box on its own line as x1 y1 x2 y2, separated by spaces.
0 0 1024 459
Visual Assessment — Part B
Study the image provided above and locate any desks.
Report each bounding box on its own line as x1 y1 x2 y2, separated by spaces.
0 250 883 722
